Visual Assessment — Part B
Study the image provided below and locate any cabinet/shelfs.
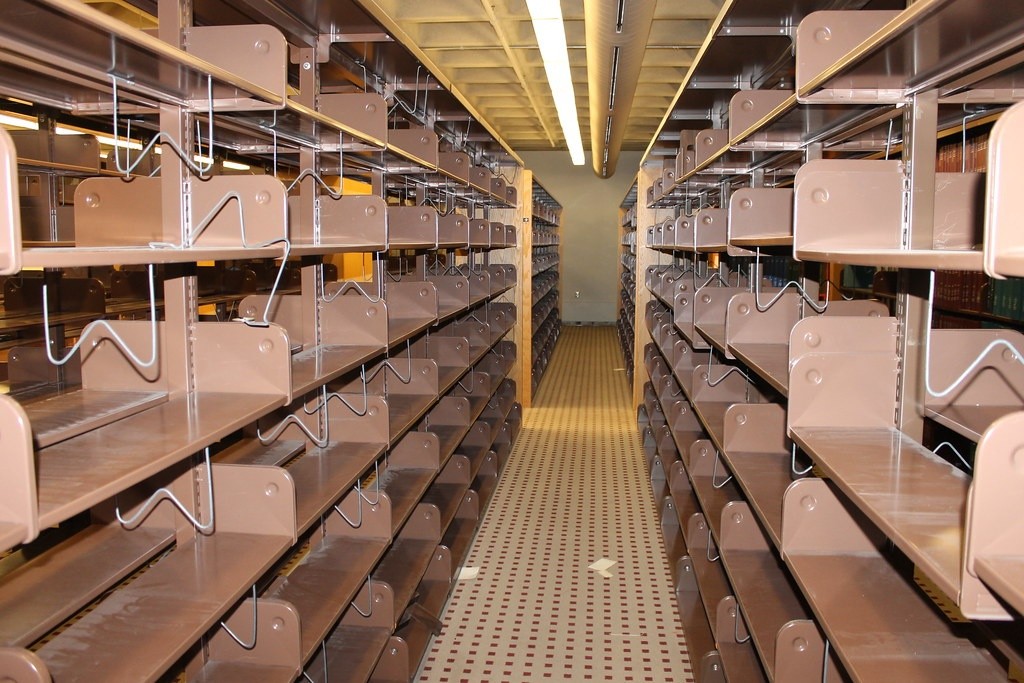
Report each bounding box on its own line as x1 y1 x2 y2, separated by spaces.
1 1 1024 683
